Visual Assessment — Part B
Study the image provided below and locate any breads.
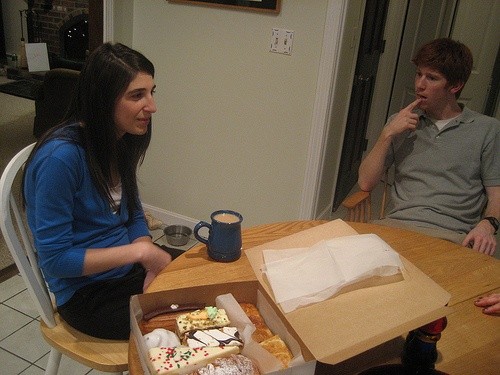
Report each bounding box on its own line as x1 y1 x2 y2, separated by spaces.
240 303 274 343
256 334 293 369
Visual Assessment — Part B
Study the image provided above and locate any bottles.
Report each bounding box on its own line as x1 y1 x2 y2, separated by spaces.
11 55 18 69
19 37 26 66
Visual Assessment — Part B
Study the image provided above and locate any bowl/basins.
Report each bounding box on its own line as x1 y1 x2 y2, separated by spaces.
163 224 192 246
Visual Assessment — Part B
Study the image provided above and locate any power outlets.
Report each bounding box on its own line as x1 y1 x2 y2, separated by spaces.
270 28 295 55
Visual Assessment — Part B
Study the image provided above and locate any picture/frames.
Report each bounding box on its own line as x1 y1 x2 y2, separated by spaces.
167 0 281 14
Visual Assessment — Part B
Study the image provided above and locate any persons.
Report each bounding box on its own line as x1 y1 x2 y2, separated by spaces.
21 43 187 339
358 38 500 255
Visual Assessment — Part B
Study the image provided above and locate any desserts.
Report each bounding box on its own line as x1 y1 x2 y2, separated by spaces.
139 307 259 375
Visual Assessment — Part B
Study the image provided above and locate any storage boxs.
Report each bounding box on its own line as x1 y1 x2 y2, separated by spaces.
130 217 454 375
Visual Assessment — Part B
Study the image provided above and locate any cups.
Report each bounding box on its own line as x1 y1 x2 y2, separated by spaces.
194 209 243 262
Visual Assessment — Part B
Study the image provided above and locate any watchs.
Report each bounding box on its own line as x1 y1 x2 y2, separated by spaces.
483 216 500 234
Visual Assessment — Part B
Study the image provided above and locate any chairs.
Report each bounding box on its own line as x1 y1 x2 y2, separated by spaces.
343 169 388 222
32 69 81 139
0 142 129 375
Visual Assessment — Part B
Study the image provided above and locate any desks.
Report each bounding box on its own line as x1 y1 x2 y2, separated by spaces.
141 220 500 375
